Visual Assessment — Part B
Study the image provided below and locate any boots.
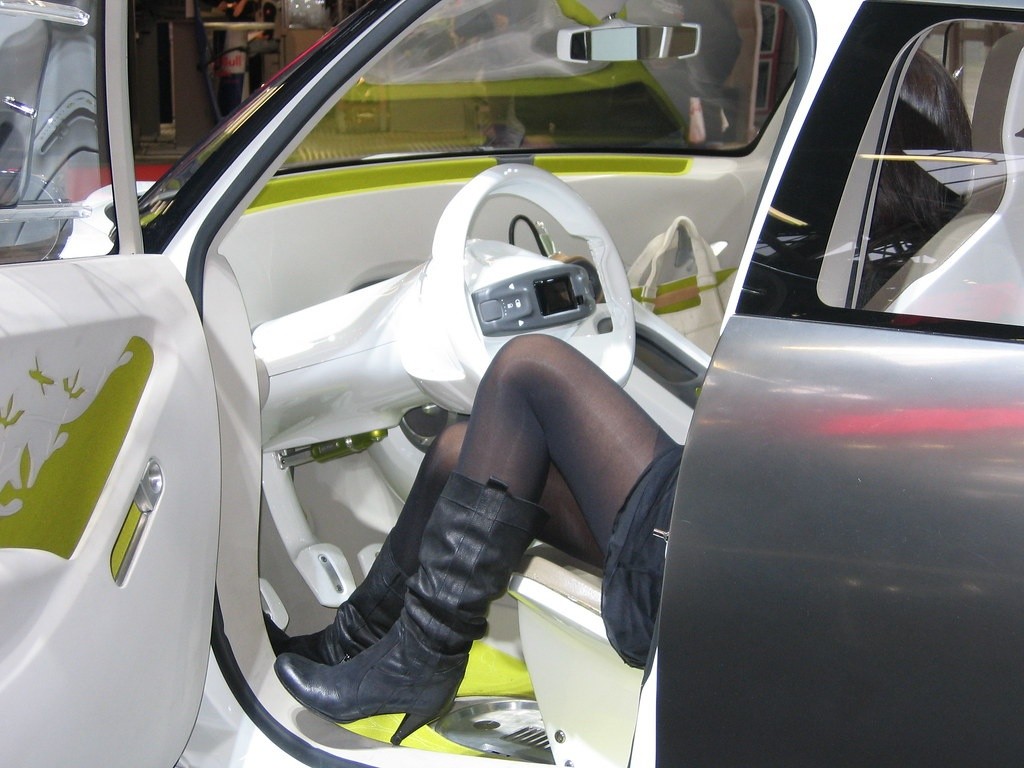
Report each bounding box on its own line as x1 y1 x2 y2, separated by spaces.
268 532 413 664
275 474 546 746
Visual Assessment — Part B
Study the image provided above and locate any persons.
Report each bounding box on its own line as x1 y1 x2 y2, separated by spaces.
273 47 974 743
217 0 740 150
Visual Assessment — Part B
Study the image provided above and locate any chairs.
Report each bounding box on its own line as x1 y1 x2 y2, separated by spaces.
503 31 1024 766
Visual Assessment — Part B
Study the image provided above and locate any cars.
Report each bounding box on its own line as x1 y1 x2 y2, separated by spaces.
0 0 1023 767
